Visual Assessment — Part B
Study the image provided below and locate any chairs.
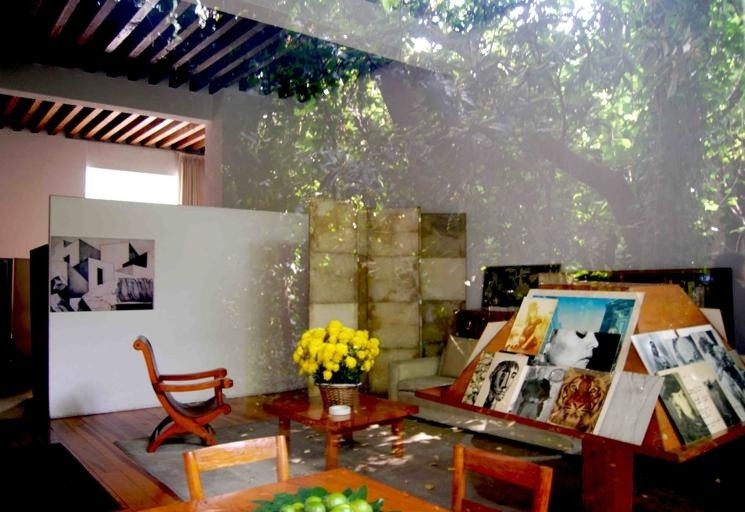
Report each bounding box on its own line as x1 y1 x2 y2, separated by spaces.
133 335 234 453
452 443 555 512
182 433 291 501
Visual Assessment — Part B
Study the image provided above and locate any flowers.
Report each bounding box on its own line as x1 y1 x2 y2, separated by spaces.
291 318 382 383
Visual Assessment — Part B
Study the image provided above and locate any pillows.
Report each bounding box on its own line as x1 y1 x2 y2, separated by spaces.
437 335 478 379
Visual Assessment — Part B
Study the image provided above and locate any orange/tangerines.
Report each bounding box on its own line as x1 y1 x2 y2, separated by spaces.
279 493 373 512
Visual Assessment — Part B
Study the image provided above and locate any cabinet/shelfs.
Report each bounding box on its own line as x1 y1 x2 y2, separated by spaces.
412 282 745 512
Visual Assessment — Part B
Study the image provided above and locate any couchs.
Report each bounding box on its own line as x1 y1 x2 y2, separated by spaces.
387 335 583 457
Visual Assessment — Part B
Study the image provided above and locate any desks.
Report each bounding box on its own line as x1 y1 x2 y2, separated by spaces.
136 466 451 512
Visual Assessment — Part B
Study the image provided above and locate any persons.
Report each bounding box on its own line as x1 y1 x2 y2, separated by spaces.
543 329 603 372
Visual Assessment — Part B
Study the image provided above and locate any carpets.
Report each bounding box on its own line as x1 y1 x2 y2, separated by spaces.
113 415 560 509
